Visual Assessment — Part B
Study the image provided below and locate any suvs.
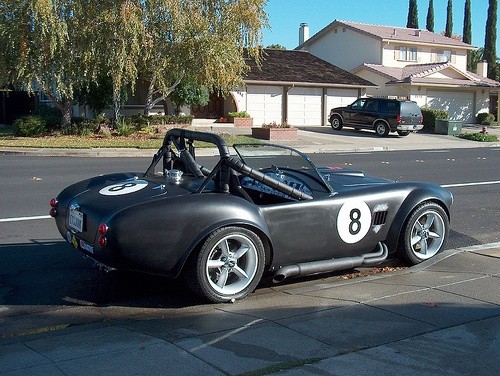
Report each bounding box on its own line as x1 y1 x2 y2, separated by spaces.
327 97 425 138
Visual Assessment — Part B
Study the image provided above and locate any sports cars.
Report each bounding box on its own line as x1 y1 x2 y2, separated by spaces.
49 127 454 305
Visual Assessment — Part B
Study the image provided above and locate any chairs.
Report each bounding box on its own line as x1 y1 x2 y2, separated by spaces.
366 103 374 112
229 174 255 204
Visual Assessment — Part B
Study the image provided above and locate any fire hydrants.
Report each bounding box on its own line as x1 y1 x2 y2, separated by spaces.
480 126 489 136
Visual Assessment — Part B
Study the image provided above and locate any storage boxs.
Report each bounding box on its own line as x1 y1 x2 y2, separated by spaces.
434 120 463 135
